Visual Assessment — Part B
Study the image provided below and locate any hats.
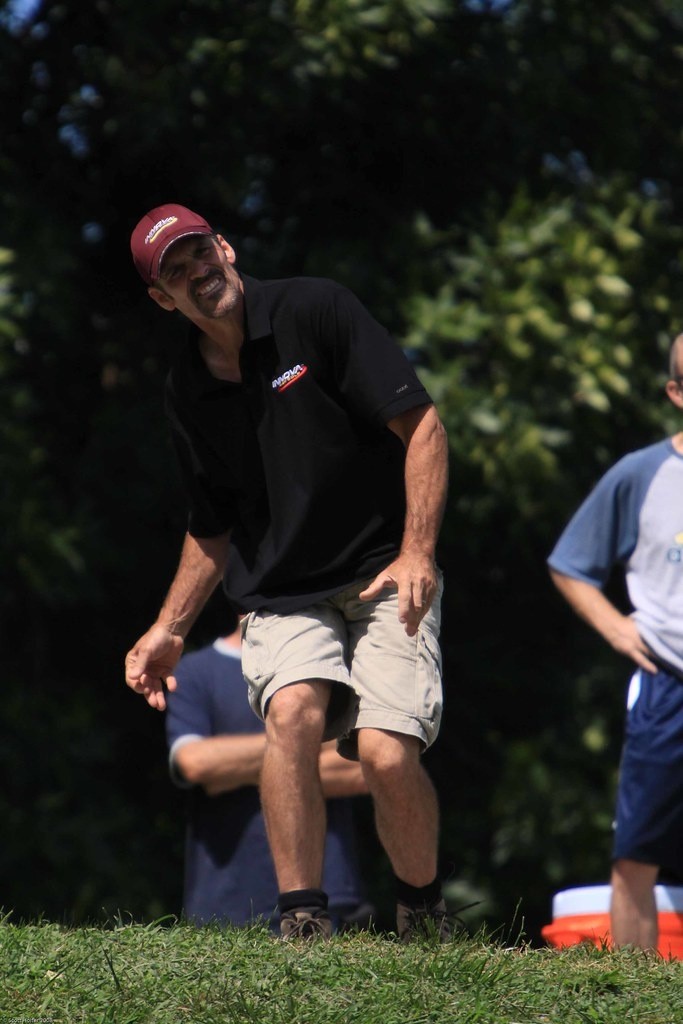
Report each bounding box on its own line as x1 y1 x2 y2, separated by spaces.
130 204 214 287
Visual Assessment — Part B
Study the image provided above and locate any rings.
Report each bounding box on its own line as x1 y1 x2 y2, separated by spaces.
422 599 427 604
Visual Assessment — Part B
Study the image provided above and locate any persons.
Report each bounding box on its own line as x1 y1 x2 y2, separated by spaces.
123 202 449 944
165 605 378 935
546 334 683 954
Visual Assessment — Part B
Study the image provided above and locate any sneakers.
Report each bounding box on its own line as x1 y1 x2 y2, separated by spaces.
279 906 333 942
397 899 451 944
